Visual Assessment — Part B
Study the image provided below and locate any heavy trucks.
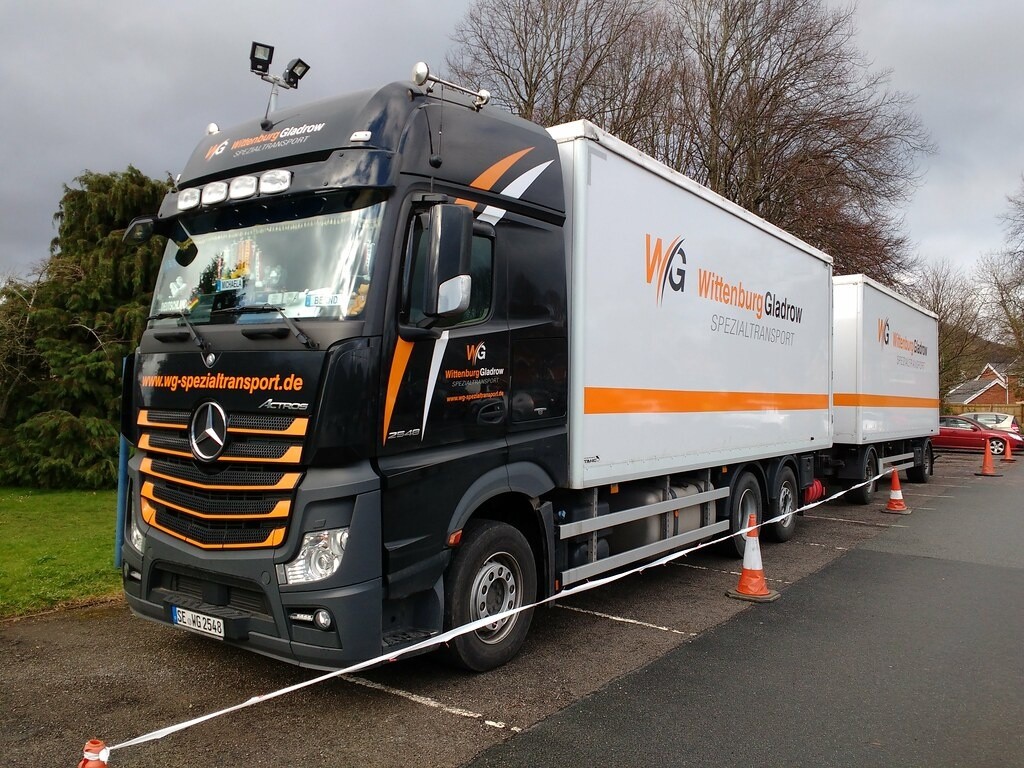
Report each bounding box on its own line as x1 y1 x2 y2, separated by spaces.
108 61 943 671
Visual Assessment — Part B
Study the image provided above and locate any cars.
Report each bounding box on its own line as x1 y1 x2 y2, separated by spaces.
929 415 1024 455
940 412 1019 434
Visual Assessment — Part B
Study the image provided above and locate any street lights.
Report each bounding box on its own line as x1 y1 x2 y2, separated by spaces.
1002 375 1008 405
248 42 310 112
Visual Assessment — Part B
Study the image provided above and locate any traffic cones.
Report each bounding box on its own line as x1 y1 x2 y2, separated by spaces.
1000 440 1016 462
878 467 913 515
79 741 109 768
974 439 1004 478
725 514 783 603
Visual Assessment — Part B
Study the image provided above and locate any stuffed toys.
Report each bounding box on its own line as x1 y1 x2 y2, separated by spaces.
350 283 370 315
